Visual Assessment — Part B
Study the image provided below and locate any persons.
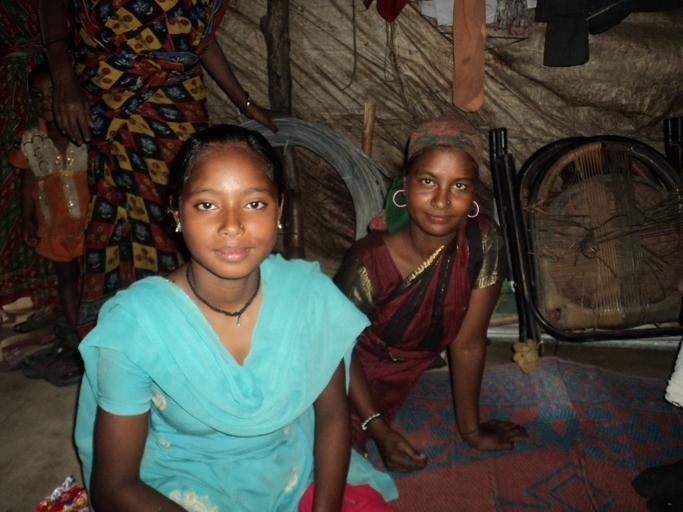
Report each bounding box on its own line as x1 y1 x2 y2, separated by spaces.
38 1 286 338
8 59 90 338
332 117 530 473
73 124 400 512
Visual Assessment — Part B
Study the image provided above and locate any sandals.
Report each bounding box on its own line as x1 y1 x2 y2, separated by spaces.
24 340 84 385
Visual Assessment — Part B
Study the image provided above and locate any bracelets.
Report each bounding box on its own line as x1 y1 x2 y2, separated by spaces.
237 91 256 119
459 427 480 435
360 413 381 431
